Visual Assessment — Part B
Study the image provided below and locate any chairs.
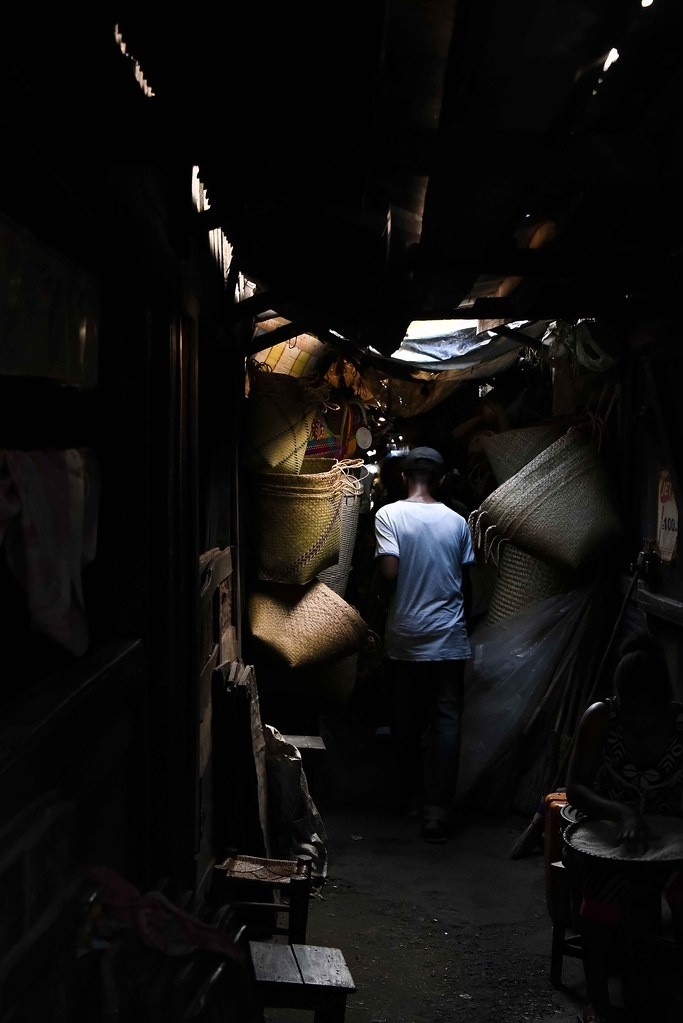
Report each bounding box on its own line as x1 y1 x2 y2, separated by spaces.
545 791 662 986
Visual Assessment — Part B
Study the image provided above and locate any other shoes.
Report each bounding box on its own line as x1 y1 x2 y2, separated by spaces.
424 822 447 843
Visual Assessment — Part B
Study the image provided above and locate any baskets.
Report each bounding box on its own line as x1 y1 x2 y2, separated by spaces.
247 362 380 705
468 425 626 630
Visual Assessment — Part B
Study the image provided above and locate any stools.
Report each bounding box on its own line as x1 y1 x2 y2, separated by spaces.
249 941 356 1023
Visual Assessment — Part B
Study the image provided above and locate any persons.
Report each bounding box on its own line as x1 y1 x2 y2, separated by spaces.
373 447 477 846
562 651 681 947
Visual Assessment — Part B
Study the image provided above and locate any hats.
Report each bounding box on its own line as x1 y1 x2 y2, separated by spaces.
404 446 444 471
